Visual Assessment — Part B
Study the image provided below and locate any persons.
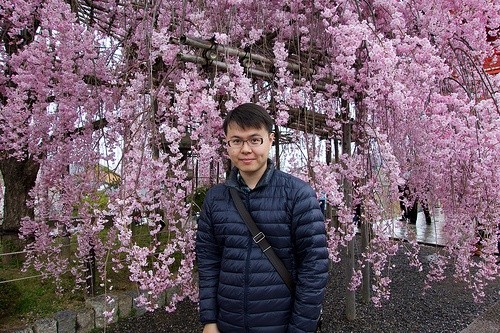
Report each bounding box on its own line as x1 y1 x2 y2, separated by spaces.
397 182 412 221
408 187 431 225
194 101 331 333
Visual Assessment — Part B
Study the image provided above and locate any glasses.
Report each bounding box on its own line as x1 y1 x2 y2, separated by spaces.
227 137 263 148
